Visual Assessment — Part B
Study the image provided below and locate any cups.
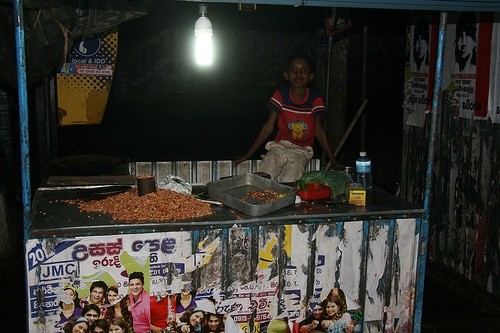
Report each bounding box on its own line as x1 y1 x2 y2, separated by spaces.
136 176 155 196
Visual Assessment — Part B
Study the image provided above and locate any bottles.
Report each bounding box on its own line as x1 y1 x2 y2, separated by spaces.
356 152 372 190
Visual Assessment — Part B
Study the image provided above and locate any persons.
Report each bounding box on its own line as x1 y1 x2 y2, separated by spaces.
56 273 354 333
234 53 355 172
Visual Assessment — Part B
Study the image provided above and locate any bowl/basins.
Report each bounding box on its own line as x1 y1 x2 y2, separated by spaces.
294 183 330 201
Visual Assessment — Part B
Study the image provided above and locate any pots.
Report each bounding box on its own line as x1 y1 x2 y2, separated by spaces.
47 145 130 177
